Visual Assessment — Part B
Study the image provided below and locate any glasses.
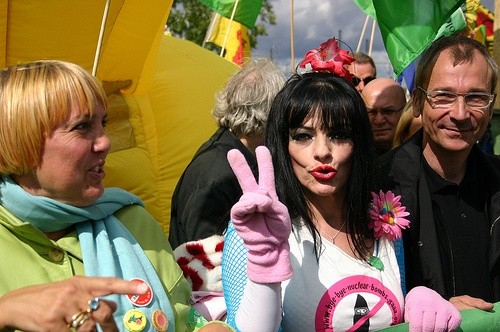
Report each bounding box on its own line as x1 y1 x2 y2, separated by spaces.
367 105 405 117
352 76 376 86
417 86 495 109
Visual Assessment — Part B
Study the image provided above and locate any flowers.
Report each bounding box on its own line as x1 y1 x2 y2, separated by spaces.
300 38 355 85
369 189 411 253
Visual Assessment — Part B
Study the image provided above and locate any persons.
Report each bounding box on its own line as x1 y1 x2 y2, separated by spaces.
1 58 237 332
167 54 289 257
219 73 461 332
370 35 500 312
343 50 424 165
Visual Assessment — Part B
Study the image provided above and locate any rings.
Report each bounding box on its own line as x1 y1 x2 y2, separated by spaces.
83 297 102 314
67 310 90 329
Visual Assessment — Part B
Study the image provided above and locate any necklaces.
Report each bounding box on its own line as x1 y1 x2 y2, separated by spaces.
321 216 346 245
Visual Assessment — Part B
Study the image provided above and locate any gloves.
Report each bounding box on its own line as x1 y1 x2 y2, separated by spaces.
404 286 462 332
227 146 294 283
